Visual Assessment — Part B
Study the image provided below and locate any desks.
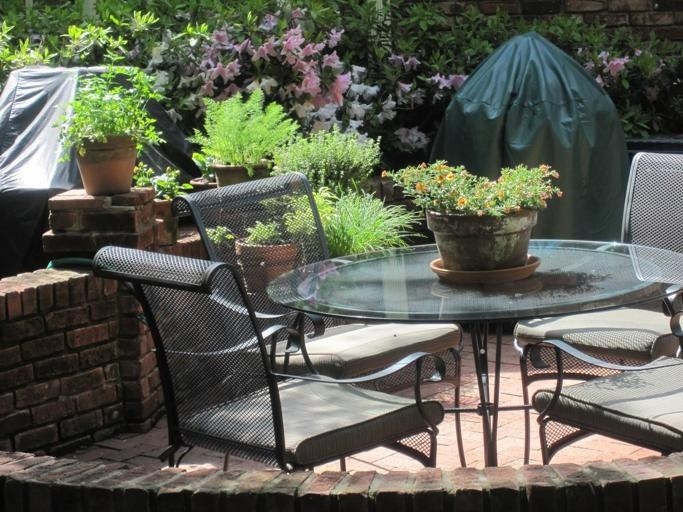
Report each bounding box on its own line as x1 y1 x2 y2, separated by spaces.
267 236 683 466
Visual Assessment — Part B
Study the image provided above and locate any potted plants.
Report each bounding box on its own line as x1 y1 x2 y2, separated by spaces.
205 227 236 266
233 220 298 293
184 89 301 213
50 52 172 194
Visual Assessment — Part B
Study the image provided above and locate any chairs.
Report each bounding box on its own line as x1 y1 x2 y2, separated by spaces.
529 312 683 463
171 170 466 470
91 246 444 470
514 151 683 462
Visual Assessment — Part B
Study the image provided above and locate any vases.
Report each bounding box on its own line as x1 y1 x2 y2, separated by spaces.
424 204 541 286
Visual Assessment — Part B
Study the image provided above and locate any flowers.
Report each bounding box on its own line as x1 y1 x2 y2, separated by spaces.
382 159 564 221
